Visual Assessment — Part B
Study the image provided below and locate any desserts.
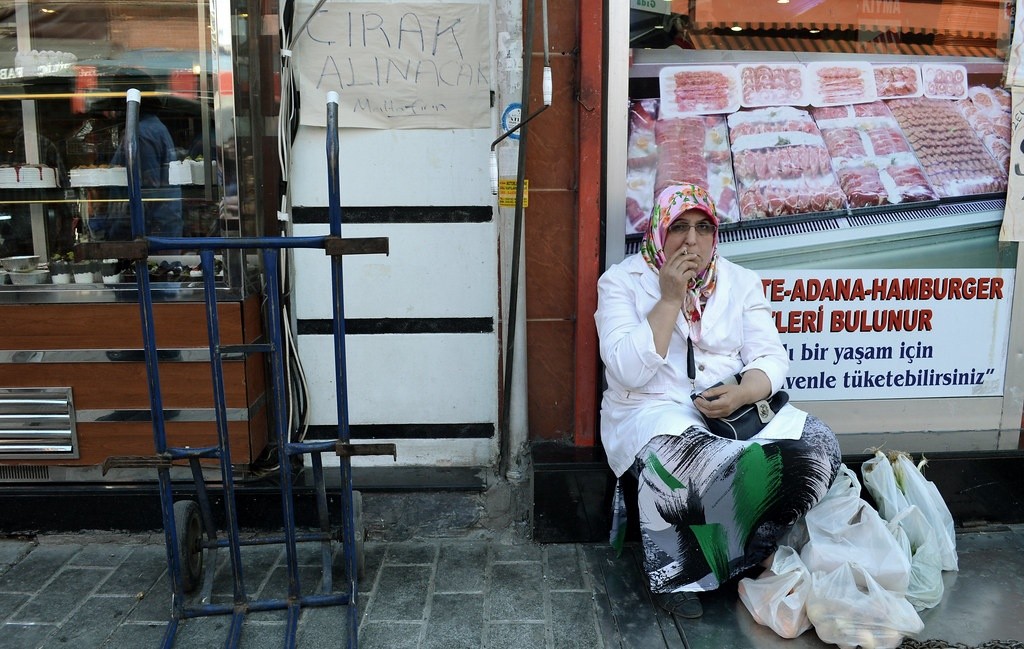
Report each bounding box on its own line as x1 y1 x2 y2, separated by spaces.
46 252 129 284
130 258 224 277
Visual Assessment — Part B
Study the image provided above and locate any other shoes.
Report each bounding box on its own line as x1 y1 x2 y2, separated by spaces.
653 592 703 618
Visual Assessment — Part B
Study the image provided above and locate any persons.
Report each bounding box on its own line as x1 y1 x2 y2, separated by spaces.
96 63 186 423
593 180 843 614
1 100 73 257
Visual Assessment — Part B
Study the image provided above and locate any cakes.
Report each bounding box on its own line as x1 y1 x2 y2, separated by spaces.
168 155 217 186
0 162 62 188
68 164 127 187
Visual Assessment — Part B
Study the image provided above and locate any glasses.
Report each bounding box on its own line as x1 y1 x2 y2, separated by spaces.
669 224 717 236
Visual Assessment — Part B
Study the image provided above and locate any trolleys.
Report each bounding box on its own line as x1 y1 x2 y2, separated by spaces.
71 88 396 648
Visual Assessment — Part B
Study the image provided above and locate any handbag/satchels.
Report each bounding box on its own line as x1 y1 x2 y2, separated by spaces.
687 336 790 440
